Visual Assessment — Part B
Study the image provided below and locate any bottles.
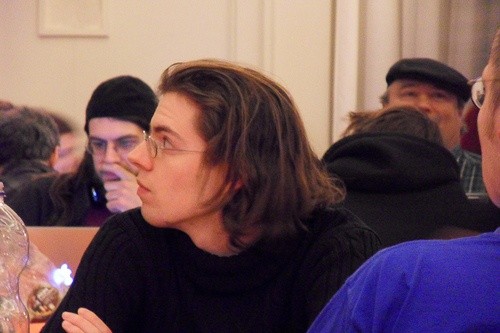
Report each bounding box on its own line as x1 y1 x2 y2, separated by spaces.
0 183 31 332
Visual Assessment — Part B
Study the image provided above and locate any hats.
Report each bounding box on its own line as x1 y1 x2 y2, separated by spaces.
386 58 470 103
85 76 159 133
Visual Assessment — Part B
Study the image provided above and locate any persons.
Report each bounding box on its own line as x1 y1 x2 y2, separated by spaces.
0 99 60 202
43 109 77 176
6 76 159 228
378 58 489 204
307 29 500 332
341 101 446 149
37 59 386 333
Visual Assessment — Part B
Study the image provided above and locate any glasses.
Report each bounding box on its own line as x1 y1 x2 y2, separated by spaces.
467 77 500 109
142 130 207 158
84 135 140 155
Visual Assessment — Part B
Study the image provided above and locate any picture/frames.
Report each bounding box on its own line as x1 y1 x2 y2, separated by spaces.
35 0 107 38
